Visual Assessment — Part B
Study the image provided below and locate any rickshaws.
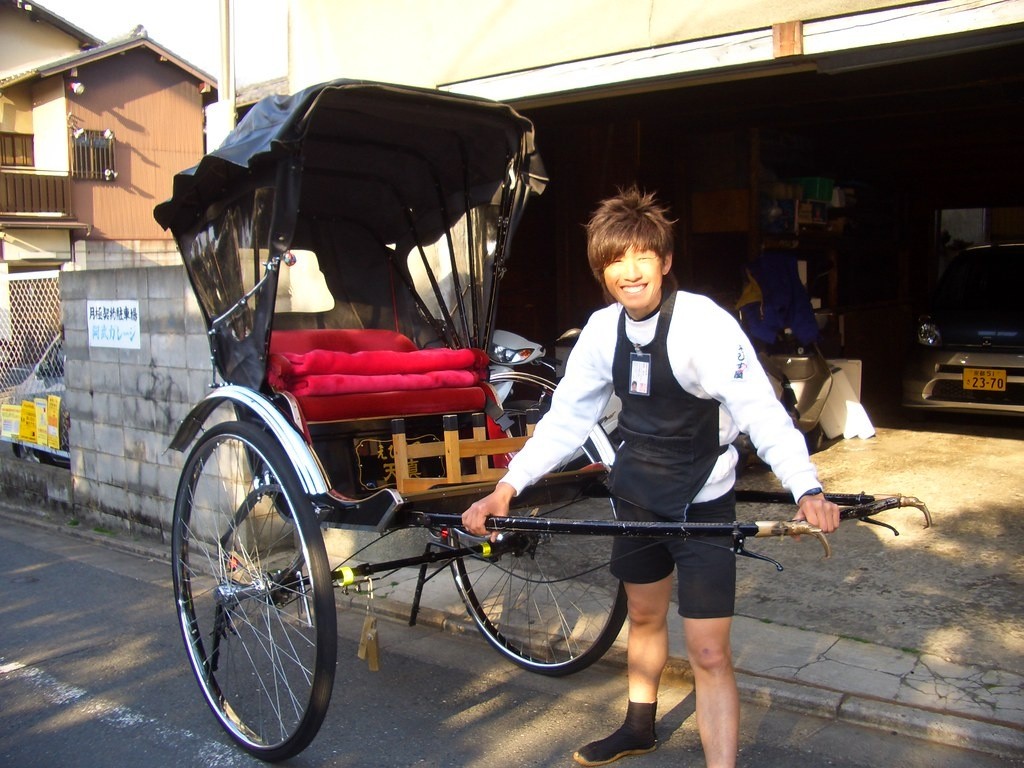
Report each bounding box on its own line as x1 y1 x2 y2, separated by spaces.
162 73 938 764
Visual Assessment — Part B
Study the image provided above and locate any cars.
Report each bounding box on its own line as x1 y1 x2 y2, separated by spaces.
0 332 72 474
899 240 1024 419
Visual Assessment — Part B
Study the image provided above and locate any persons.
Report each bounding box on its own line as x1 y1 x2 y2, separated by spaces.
462 188 839 768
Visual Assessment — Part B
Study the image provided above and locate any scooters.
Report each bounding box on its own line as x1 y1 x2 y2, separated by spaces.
484 325 623 497
716 259 835 477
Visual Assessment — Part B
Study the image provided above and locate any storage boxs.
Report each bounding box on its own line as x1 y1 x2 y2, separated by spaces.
785 176 834 201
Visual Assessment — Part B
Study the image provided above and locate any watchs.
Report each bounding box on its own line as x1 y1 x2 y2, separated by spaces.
796 487 823 500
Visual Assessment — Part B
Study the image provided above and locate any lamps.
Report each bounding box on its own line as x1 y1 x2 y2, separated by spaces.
68 81 85 96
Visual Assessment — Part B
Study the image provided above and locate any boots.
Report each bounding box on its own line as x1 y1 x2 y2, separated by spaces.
573 699 659 766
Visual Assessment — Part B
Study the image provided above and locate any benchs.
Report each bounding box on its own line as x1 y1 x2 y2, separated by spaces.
252 329 489 425
